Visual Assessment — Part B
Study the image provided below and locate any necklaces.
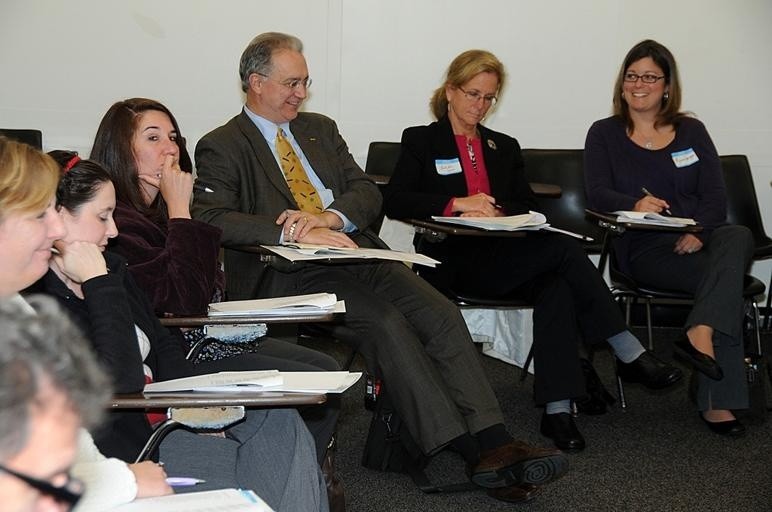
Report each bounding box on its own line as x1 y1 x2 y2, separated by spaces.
634 127 666 150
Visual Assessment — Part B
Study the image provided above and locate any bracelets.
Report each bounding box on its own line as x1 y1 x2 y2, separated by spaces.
290 222 296 244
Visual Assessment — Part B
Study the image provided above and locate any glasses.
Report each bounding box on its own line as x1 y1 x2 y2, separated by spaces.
1 464 87 511
457 84 498 108
623 72 666 84
257 73 313 91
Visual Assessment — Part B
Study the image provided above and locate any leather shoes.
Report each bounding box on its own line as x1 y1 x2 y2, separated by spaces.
464 459 543 505
470 440 570 488
540 405 586 452
668 333 724 381
614 350 684 390
697 409 746 436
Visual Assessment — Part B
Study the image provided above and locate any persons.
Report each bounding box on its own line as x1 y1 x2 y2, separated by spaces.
383 50 684 452
23 149 329 512
89 98 341 467
0 296 117 512
584 40 754 437
0 136 175 512
190 31 570 501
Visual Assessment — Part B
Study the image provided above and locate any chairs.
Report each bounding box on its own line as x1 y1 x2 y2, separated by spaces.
1 129 771 510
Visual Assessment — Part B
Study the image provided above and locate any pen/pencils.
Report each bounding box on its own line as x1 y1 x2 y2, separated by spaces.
284 210 289 219
157 173 215 193
477 189 503 210
166 476 207 487
49 248 110 271
642 187 672 215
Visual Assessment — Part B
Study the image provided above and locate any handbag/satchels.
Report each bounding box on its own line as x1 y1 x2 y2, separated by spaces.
361 384 407 474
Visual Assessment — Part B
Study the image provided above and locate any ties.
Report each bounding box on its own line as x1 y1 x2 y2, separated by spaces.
275 127 324 214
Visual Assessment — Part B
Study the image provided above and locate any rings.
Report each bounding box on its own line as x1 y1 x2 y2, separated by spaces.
302 217 309 222
